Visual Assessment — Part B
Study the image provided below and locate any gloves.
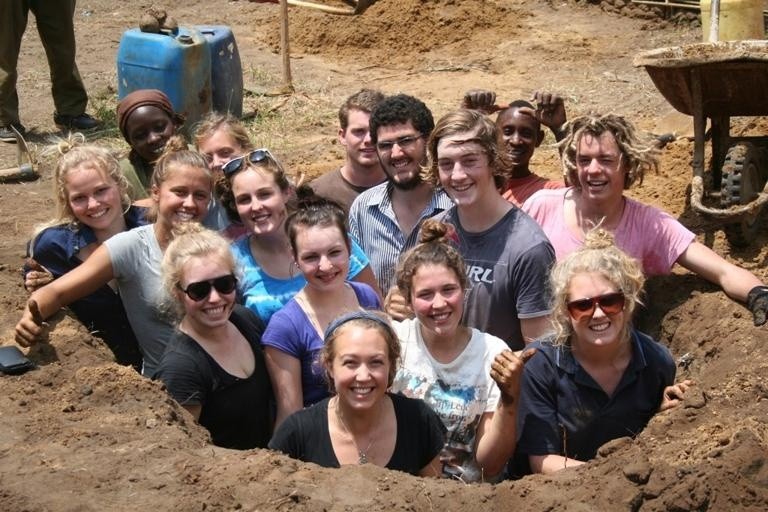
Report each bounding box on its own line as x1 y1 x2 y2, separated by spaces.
747 285 768 326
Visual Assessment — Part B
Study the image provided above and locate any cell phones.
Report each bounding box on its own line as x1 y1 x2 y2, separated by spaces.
0 346 33 375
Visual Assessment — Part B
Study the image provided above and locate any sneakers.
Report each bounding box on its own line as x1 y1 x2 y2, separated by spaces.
53 110 97 132
0 125 25 142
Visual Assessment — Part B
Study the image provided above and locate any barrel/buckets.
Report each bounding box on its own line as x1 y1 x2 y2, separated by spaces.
193 25 241 117
117 28 212 116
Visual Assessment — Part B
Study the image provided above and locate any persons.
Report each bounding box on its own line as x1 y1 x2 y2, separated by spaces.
268 308 448 479
504 226 691 475
149 219 271 450
261 185 385 441
0 0 100 142
391 218 537 484
14 90 768 380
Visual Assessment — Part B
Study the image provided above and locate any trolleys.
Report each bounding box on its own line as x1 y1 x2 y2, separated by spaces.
631 0 768 245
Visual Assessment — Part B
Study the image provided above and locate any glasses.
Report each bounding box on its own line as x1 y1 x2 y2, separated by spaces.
177 271 238 301
377 135 423 148
221 148 282 176
567 292 625 321
